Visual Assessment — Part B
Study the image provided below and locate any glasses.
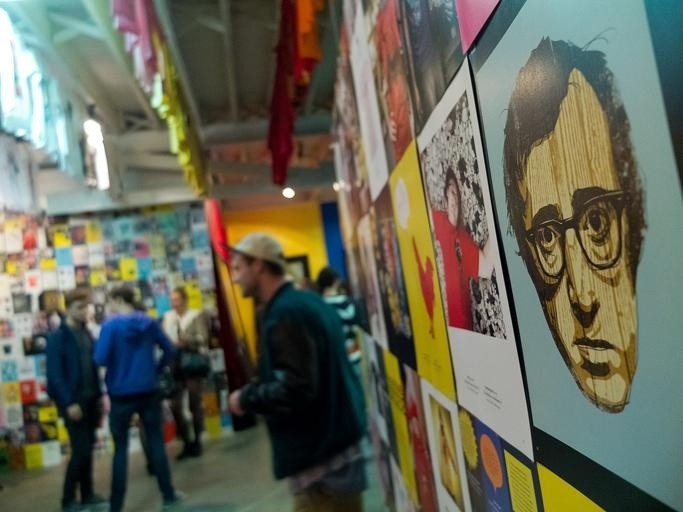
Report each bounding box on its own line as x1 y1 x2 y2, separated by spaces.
523 190 630 279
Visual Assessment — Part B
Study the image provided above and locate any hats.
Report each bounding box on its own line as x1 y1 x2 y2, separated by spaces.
220 232 286 269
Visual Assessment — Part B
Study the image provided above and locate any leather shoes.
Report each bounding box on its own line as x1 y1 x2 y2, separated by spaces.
176 442 200 460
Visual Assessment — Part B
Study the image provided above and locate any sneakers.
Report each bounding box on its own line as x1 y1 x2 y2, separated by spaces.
64 494 108 512
162 491 186 509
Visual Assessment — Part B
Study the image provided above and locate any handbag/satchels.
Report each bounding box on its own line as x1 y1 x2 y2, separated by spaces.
179 342 209 377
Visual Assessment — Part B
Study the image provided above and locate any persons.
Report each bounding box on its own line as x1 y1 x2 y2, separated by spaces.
503 36 646 414
314 264 362 375
45 285 211 511
217 232 369 512
421 159 495 331
